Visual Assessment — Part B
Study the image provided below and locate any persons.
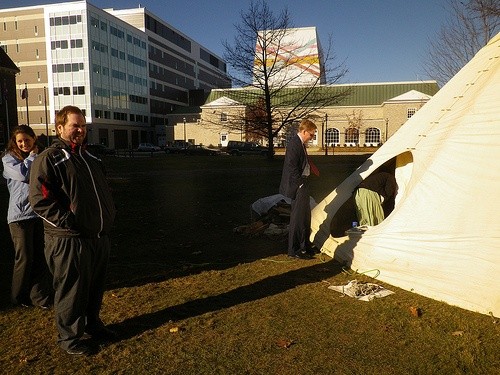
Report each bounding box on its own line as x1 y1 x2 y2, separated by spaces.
278 119 318 260
27 105 118 355
128 141 240 157
1 124 54 310
351 162 396 226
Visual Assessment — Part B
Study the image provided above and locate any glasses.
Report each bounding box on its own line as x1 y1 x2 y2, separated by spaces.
306 130 314 138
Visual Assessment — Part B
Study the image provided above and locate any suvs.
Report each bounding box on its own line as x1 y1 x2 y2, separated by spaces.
136 143 160 152
227 142 275 157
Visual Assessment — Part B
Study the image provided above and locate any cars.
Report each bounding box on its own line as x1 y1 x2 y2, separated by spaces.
163 142 196 153
183 146 221 156
87 144 113 154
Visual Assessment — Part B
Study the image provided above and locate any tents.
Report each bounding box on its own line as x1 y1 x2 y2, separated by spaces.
309 32 500 319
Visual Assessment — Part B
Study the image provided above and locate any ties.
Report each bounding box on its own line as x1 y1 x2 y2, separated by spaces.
303 144 320 177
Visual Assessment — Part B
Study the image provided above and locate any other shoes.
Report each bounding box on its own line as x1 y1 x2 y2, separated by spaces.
41 303 51 310
293 249 311 259
86 326 118 341
304 244 320 254
66 343 88 355
19 303 32 309
